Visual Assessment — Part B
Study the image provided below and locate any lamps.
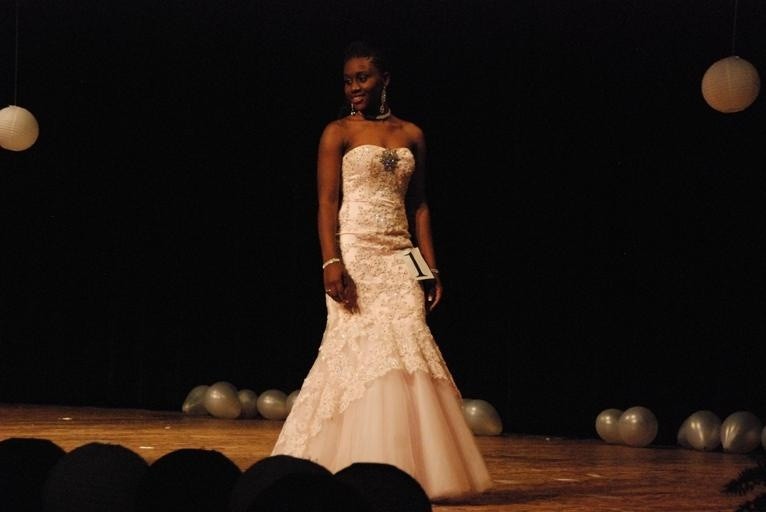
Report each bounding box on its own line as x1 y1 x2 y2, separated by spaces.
0 0 39 151
700 0 758 113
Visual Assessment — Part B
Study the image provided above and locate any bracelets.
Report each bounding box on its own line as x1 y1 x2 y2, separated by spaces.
322 257 340 271
431 268 440 274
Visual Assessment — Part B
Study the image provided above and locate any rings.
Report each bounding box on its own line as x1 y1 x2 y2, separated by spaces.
325 289 331 292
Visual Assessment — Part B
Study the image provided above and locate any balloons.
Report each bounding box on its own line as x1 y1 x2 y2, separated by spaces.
594 406 766 456
461 397 506 436
181 380 301 422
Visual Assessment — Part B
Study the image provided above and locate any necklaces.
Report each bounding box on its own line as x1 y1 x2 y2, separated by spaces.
357 106 392 120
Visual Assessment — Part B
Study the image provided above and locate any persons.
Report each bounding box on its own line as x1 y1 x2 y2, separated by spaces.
269 34 498 506
